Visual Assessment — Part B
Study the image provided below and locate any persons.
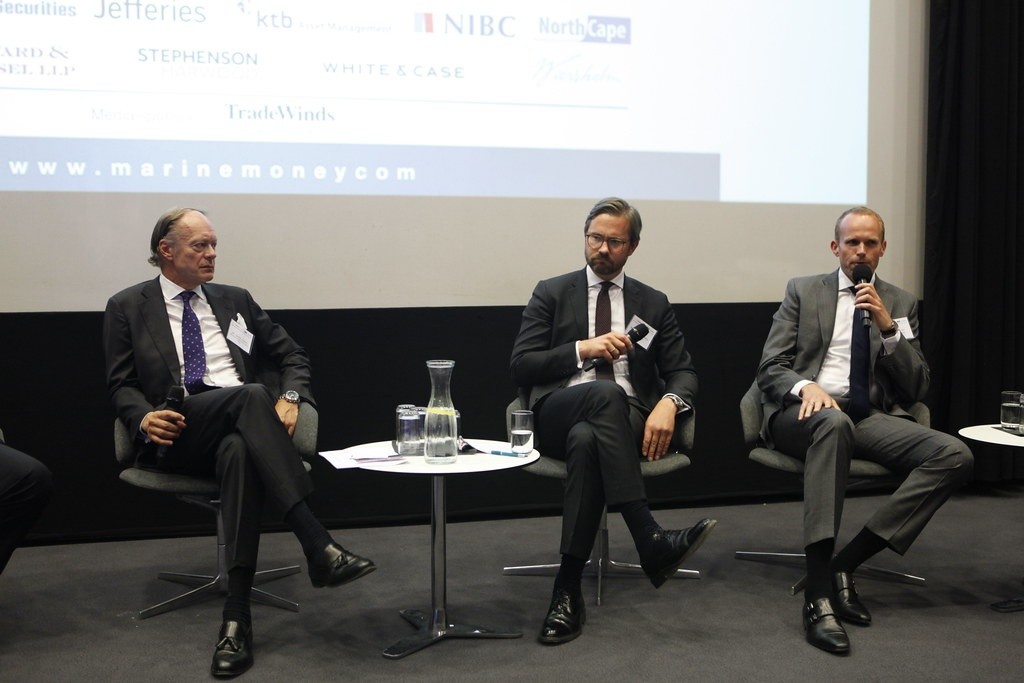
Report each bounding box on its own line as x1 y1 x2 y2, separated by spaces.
101 208 376 677
509 197 718 646
0 430 53 575
756 206 974 654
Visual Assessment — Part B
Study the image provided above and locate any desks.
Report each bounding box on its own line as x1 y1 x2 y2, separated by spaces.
343 438 541 660
957 424 1024 612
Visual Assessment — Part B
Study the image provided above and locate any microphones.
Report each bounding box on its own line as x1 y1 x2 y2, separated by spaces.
164 386 184 424
852 265 873 327
584 323 649 371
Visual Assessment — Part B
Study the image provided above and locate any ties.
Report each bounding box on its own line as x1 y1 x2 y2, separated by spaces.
848 286 870 425
178 291 222 395
595 281 616 382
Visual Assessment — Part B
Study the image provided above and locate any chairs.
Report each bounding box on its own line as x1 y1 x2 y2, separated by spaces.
114 399 319 621
734 376 932 596
503 397 701 606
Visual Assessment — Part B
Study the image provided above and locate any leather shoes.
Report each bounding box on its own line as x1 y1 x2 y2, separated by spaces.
802 597 850 653
640 517 717 589
210 620 254 678
305 540 377 589
833 570 872 623
539 593 585 643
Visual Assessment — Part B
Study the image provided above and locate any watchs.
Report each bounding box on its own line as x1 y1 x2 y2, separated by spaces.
279 390 300 405
880 321 898 335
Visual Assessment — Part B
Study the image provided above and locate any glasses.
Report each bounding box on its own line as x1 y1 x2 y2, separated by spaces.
585 233 632 254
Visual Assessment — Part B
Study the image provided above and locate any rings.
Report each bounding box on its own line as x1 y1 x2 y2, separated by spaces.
611 348 615 352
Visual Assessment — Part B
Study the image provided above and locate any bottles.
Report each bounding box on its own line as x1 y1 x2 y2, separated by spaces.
424 360 458 464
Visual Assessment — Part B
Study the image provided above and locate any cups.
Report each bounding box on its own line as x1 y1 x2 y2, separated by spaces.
395 404 461 455
1000 391 1024 435
510 410 533 453
